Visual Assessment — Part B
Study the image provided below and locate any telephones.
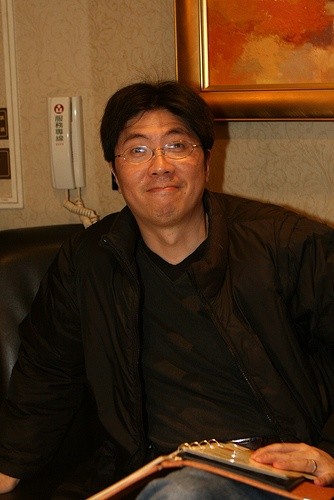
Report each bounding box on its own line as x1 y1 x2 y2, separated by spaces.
46 95 85 190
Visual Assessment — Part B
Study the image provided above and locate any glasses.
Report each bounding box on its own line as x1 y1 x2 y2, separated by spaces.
113 140 205 165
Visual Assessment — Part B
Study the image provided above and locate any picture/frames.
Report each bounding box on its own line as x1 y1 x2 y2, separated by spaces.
174 0 334 124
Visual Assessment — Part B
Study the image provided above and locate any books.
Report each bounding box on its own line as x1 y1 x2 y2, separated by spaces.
86 439 334 500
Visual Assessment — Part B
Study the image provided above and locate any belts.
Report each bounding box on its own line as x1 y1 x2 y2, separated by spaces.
143 436 282 452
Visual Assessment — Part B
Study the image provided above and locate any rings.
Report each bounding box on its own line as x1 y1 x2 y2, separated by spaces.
311 459 317 474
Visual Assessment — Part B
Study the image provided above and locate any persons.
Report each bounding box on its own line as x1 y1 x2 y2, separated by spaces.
0 79 334 500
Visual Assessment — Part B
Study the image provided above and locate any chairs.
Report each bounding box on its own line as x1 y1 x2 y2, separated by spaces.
0 223 87 386
